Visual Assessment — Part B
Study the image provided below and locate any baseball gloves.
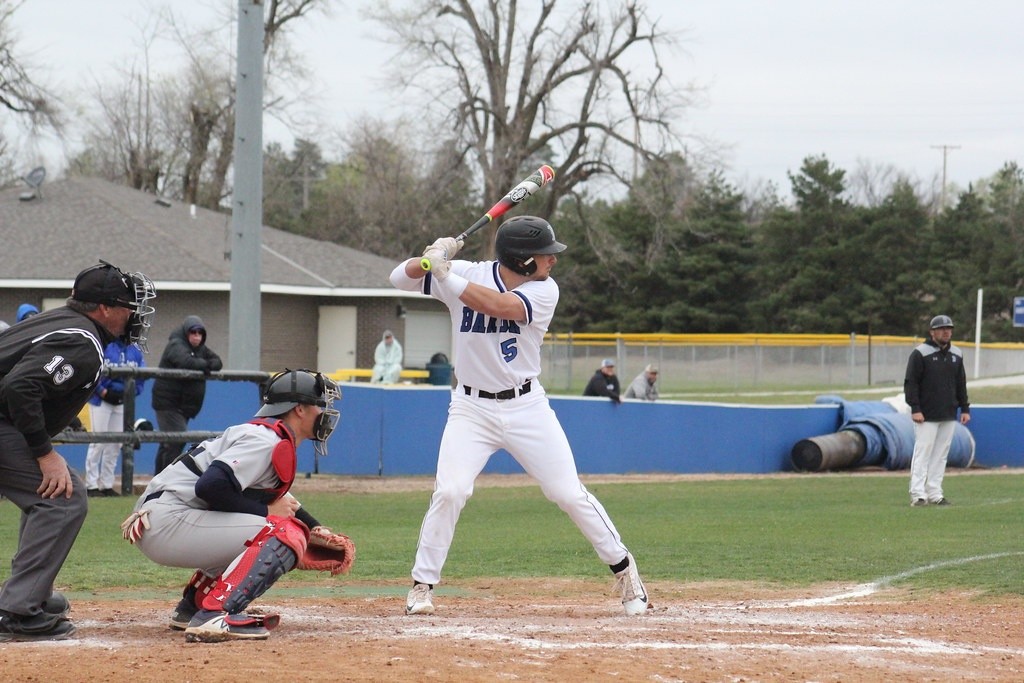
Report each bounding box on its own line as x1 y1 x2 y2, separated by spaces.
296 525 358 575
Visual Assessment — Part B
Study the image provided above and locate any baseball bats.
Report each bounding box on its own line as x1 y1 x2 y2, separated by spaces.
421 164 555 271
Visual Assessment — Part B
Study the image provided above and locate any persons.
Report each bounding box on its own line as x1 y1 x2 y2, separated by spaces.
583 359 624 404
0 259 156 643
388 215 650 617
904 315 970 508
16 303 40 323
370 330 403 384
86 341 148 498
624 364 659 401
152 315 222 475
134 367 356 641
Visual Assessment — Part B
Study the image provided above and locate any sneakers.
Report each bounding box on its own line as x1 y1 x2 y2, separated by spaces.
184 610 270 642
0 613 76 640
168 600 199 629
931 498 951 505
911 498 929 507
403 584 434 614
43 594 70 615
141 490 164 507
611 551 649 614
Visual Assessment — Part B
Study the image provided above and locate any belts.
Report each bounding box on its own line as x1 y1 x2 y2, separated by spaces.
464 381 531 399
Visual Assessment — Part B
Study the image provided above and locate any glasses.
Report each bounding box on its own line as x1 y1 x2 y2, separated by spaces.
191 330 203 335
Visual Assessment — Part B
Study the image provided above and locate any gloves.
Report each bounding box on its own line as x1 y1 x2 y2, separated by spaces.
422 246 453 283
422 238 464 259
119 510 150 544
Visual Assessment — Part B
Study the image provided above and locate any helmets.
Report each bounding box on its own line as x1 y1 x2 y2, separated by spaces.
254 370 316 416
930 315 954 329
495 216 568 276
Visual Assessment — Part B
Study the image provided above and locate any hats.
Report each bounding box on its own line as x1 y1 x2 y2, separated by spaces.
646 364 660 372
601 359 615 367
75 265 135 301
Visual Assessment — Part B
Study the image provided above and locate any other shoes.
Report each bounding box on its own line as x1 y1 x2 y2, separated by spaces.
87 488 120 497
